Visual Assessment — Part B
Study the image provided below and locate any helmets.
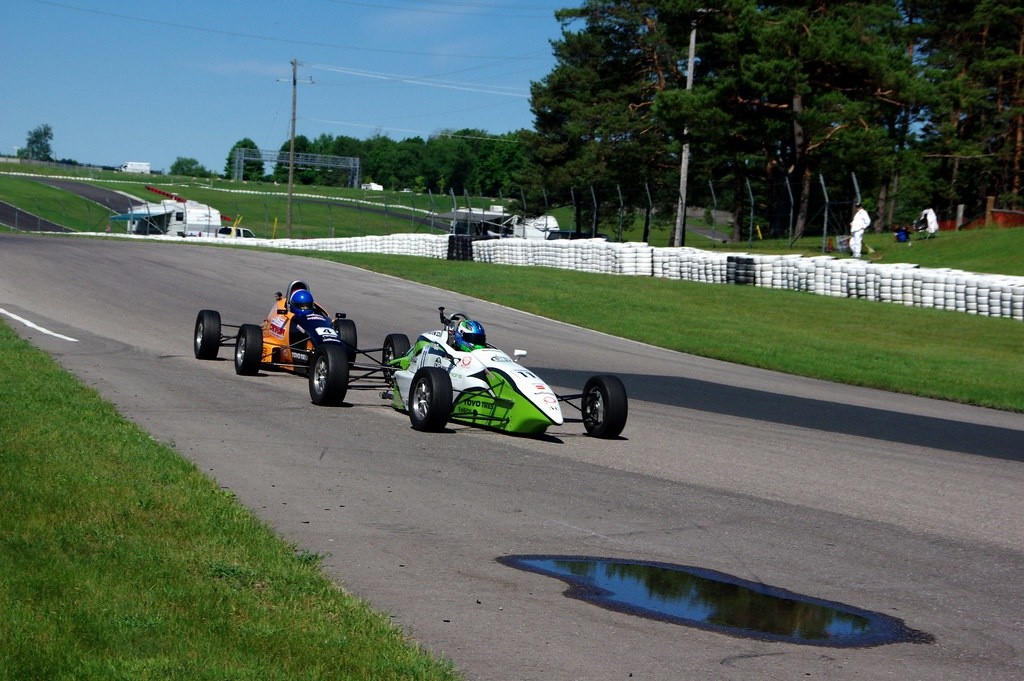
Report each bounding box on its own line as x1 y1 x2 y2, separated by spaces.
454 319 486 351
289 287 314 315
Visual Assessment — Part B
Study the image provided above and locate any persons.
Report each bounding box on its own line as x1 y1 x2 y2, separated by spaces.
848 202 871 258
451 320 487 353
893 223 910 243
290 289 314 317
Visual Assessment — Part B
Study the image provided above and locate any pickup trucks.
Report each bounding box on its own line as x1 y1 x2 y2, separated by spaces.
198 226 255 240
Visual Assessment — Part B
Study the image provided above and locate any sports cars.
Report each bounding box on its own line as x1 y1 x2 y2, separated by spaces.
194 280 410 385
309 306 628 439
